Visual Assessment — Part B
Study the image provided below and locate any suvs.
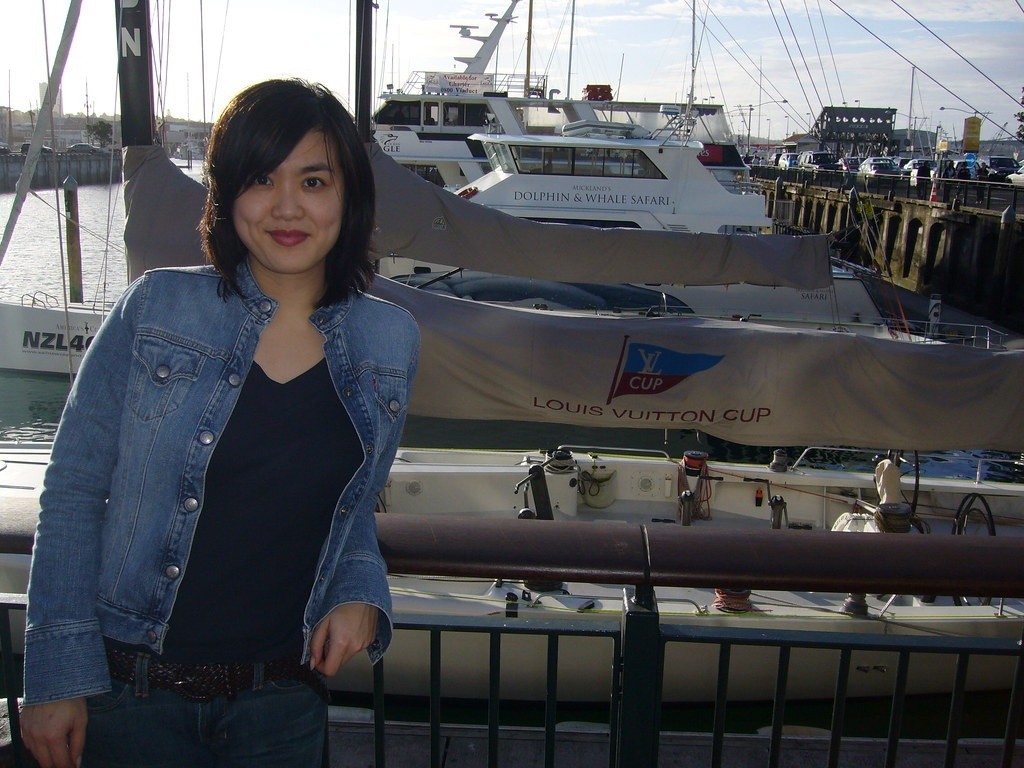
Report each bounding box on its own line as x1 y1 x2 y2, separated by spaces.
797 151 837 171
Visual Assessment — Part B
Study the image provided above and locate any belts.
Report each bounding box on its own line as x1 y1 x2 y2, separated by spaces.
100 647 334 705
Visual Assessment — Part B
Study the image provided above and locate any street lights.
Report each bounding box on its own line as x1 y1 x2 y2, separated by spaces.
938 106 993 117
746 99 788 153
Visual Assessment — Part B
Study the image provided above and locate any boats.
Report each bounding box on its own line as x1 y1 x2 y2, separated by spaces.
0 0 1022 704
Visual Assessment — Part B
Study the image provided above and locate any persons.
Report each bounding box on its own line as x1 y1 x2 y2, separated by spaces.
19 79 422 768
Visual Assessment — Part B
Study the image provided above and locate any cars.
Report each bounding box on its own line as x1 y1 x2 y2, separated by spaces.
836 156 1021 182
767 152 798 168
